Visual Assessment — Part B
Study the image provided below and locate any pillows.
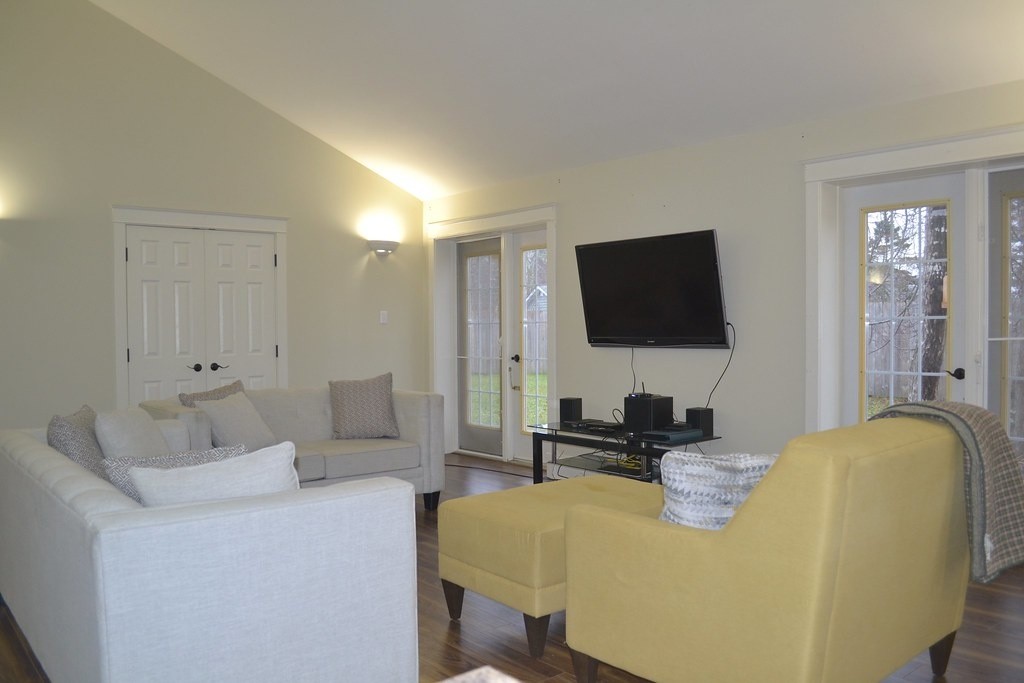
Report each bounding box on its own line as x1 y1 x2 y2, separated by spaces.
128 441 300 508
657 450 779 531
178 379 246 408
194 391 278 454
94 406 170 458
328 372 400 441
99 443 248 505
46 404 111 482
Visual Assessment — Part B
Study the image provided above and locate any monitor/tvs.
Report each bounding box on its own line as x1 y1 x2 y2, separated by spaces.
575 228 730 350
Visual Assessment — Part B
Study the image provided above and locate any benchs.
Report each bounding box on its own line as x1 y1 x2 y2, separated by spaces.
437 473 665 660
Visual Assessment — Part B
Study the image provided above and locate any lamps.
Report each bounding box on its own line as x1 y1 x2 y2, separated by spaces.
367 239 400 257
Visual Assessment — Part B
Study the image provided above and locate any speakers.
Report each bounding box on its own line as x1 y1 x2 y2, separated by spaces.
560 397 583 431
686 407 713 440
624 396 673 435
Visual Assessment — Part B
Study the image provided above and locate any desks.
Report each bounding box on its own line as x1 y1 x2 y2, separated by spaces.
527 422 723 485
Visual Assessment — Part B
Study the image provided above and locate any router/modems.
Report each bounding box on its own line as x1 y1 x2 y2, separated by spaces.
629 382 660 398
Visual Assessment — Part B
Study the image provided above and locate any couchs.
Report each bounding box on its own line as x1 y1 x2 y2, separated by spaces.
139 387 445 511
565 400 1024 683
0 419 420 683
